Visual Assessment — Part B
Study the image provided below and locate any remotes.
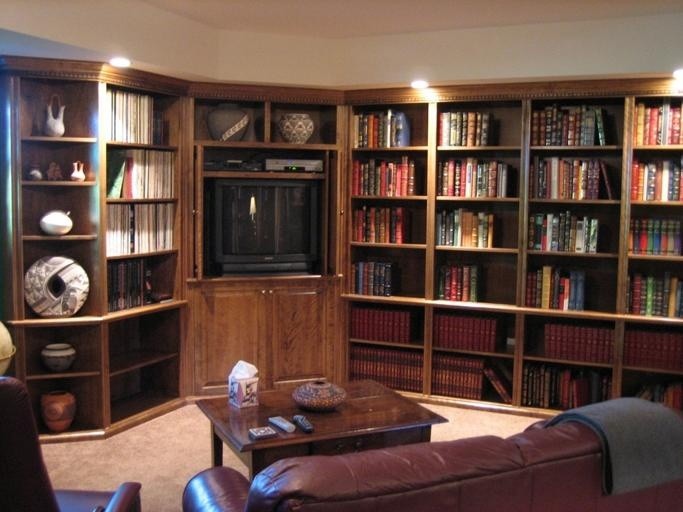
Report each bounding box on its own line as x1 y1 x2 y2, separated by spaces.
268 415 296 433
293 414 313 432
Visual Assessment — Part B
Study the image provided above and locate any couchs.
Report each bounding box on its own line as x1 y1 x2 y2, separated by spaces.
183 405 683 511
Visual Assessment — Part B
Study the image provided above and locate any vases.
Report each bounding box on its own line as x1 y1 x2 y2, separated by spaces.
278 112 314 144
206 102 251 141
292 380 347 410
40 343 77 373
40 391 77 432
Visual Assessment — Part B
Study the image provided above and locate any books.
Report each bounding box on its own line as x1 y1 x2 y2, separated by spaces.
430 109 514 404
105 86 177 313
346 109 426 395
520 101 617 411
621 99 683 416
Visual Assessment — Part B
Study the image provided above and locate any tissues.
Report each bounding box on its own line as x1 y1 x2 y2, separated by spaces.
228 360 259 408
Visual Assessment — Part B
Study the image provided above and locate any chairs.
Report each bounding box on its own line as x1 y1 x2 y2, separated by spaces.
1 376 142 511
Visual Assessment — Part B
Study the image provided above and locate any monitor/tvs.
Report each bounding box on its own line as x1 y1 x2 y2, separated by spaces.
210 178 319 273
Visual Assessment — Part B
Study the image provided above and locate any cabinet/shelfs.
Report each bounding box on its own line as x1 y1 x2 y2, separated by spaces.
194 283 328 388
341 98 682 421
0 54 187 442
